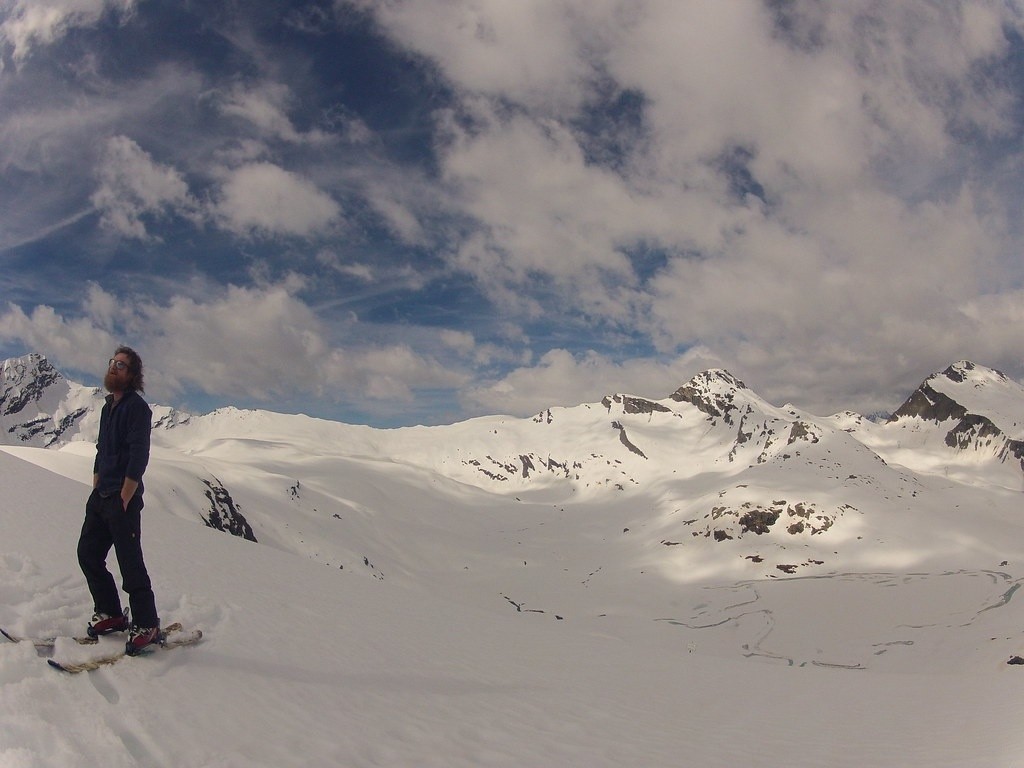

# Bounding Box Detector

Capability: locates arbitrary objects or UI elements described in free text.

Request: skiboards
[0,622,202,674]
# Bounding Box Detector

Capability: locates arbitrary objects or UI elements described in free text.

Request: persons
[77,345,159,649]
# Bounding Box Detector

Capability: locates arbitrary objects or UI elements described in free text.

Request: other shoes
[89,613,160,648]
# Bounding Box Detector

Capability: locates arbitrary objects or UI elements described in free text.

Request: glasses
[109,358,131,370]
